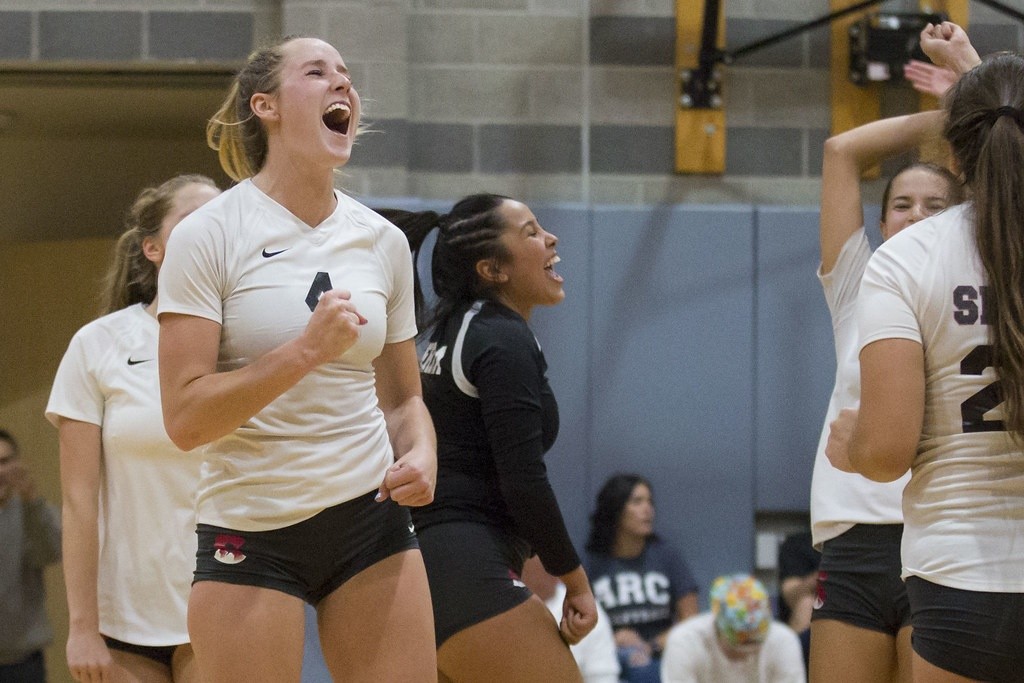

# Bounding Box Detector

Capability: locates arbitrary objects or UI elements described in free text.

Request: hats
[711,573,772,655]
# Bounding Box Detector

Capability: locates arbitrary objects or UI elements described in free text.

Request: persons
[659,571,806,683]
[155,37,440,682]
[518,548,620,683]
[775,528,822,683]
[41,174,220,683]
[824,47,1024,682]
[0,430,62,683]
[582,469,699,683]
[376,187,595,682]
[809,21,981,683]
